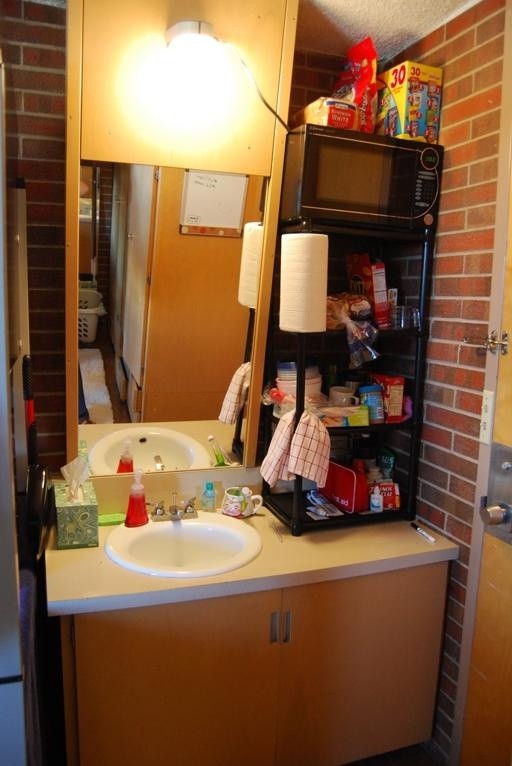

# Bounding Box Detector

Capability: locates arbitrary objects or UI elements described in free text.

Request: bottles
[201,482,217,513]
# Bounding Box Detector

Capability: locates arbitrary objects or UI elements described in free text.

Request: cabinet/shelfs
[267,227,434,541]
[111,164,267,422]
[62,559,448,763]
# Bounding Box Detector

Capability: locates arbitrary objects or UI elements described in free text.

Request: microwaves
[279,123,445,232]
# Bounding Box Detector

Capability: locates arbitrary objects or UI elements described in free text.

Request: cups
[221,485,263,519]
[329,386,360,406]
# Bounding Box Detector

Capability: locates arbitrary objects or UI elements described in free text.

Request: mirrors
[81,161,279,474]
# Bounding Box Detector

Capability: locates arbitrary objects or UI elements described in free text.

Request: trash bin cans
[78,272,103,343]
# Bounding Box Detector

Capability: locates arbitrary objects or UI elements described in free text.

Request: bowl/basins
[274,361,323,399]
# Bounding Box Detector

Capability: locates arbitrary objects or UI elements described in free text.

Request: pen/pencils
[410,520,436,543]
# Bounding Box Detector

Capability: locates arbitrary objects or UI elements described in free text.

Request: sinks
[106,509,264,578]
[90,426,211,475]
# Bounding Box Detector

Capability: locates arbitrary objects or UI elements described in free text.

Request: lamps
[125,20,259,158]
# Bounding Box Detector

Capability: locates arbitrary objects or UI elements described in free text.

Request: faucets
[154,455,165,470]
[170,505,185,521]
